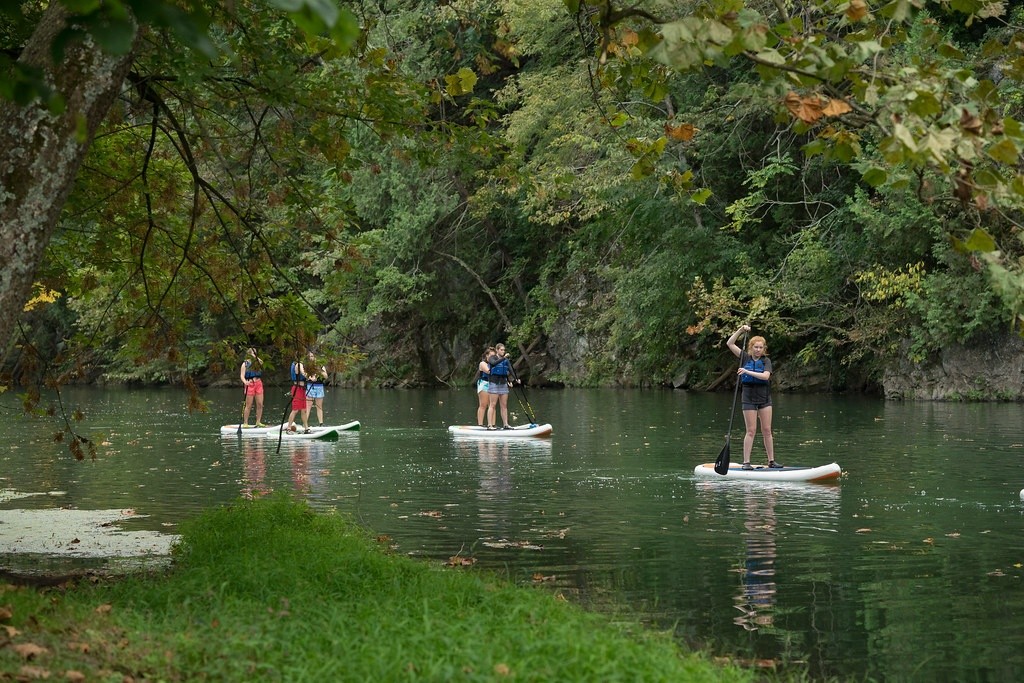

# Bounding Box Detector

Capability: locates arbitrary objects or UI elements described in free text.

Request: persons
[725,325,784,470]
[285,350,329,437]
[239,347,266,430]
[476,343,521,431]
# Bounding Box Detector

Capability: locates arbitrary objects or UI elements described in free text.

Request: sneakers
[768,460,783,468]
[742,463,753,470]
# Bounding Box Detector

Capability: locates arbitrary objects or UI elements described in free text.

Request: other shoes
[244,424,249,428]
[286,429,295,435]
[480,424,484,427]
[319,423,326,426]
[256,422,265,427]
[493,426,501,430]
[304,429,313,434]
[503,425,514,429]
[487,425,497,430]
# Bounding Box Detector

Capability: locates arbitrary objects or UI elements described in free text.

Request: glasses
[499,348,506,351]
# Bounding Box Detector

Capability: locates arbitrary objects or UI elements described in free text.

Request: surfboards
[447,423,555,438]
[694,462,842,482]
[220,420,361,434]
[266,430,339,438]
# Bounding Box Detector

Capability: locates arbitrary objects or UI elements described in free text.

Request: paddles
[506,358,537,426]
[714,330,749,476]
